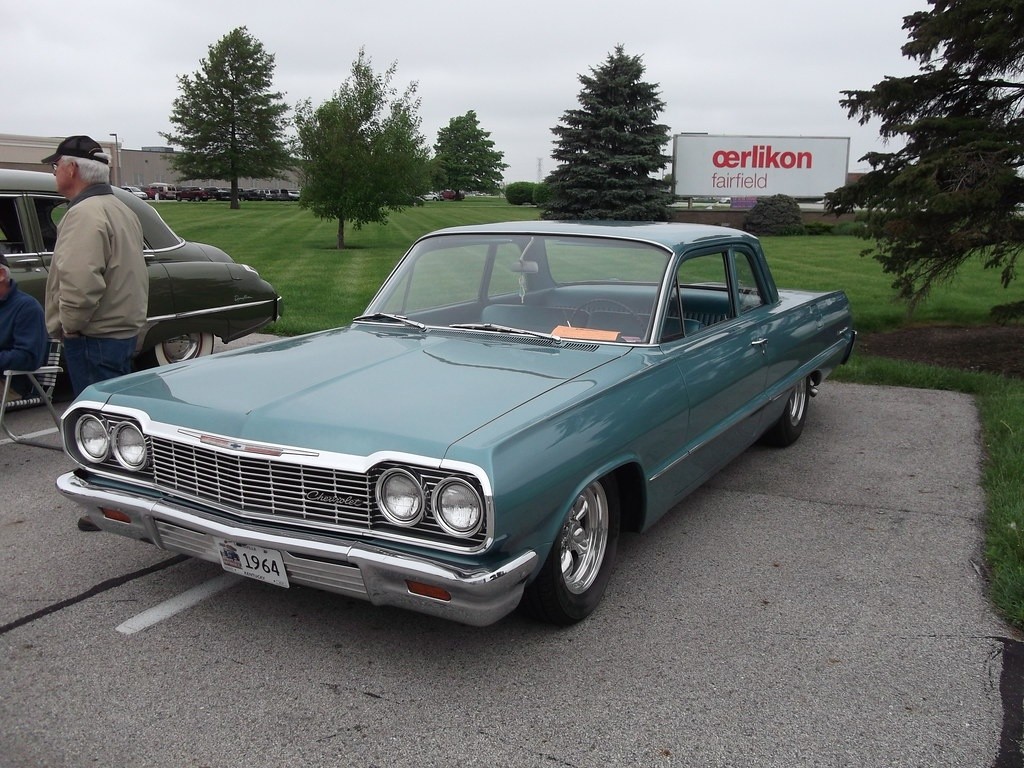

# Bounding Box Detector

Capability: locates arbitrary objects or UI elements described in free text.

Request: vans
[148,182,177,199]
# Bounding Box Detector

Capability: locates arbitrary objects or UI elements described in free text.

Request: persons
[0,253,49,403]
[41,135,149,532]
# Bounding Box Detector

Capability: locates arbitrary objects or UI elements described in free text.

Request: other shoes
[77,515,101,531]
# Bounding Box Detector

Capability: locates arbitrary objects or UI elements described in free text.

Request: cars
[422,191,445,202]
[0,167,283,368]
[175,188,215,202]
[136,185,158,199]
[56,219,860,630]
[719,198,731,203]
[176,185,300,201]
[121,185,148,199]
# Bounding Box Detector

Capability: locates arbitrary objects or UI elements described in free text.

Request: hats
[41,135,109,164]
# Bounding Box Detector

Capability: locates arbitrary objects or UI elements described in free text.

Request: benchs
[667,312,727,326]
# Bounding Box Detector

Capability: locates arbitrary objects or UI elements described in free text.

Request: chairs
[481,304,590,334]
[0,339,65,451]
[587,312,703,338]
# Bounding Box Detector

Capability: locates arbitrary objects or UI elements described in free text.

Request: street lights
[109,133,121,188]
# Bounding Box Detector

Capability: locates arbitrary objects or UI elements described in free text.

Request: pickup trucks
[441,191,465,201]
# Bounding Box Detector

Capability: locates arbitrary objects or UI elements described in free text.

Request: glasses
[51,163,67,171]
